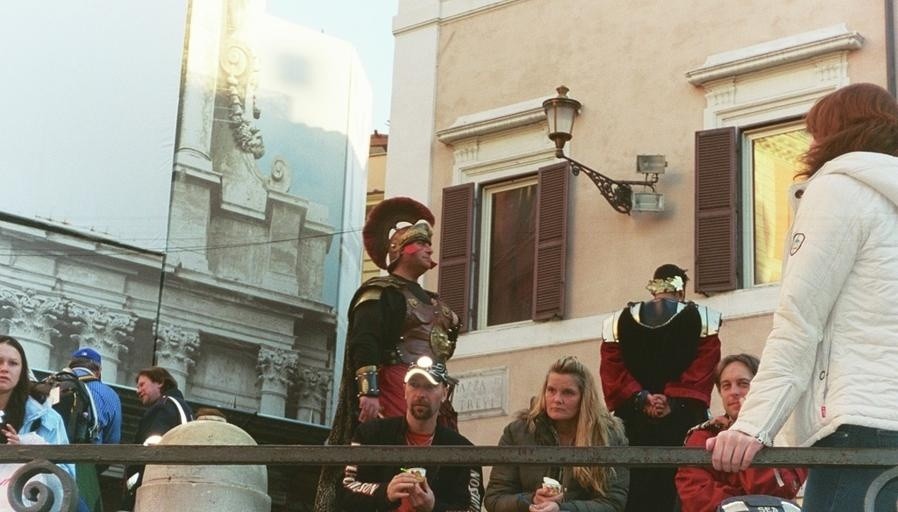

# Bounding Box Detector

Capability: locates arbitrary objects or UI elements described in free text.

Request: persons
[598,263,723,512]
[0,334,93,512]
[334,354,486,512]
[706,82,898,512]
[114,366,194,512]
[40,347,122,512]
[310,195,463,512]
[674,353,807,512]
[481,350,631,512]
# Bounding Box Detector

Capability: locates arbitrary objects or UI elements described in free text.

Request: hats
[404,359,448,387]
[72,348,101,363]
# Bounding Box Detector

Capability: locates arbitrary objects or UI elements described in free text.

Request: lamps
[542,81,667,218]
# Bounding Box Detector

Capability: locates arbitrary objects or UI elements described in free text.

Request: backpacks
[29,368,100,444]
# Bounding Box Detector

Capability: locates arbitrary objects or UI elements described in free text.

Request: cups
[406,467,427,484]
[543,482,561,494]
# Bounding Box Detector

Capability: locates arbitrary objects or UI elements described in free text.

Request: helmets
[363,197,435,273]
